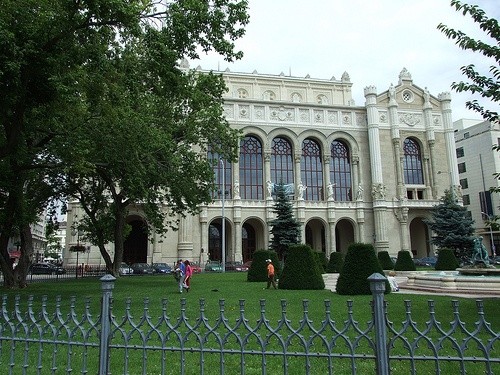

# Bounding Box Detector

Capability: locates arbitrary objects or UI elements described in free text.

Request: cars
[32,262,66,275]
[414,256,500,267]
[120,261,250,276]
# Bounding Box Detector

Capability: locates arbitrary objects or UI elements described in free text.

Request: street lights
[71,230,84,277]
[219,156,225,272]
[481,211,496,257]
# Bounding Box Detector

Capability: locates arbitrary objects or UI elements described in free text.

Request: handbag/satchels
[269,274,274,279]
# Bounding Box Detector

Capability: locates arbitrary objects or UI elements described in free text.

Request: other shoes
[265,288,269,290]
[274,288,277,290]
[177,292,182,294]
[186,287,190,292]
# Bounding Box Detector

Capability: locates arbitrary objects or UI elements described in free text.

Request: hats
[389,271,396,276]
[265,259,272,263]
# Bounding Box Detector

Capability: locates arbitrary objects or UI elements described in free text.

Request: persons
[357,183,363,199]
[51,260,64,266]
[297,181,307,198]
[326,182,335,199]
[266,180,273,197]
[371,183,387,199]
[457,184,464,200]
[403,183,406,197]
[265,259,277,290]
[473,236,484,261]
[234,180,240,198]
[174,259,192,294]
